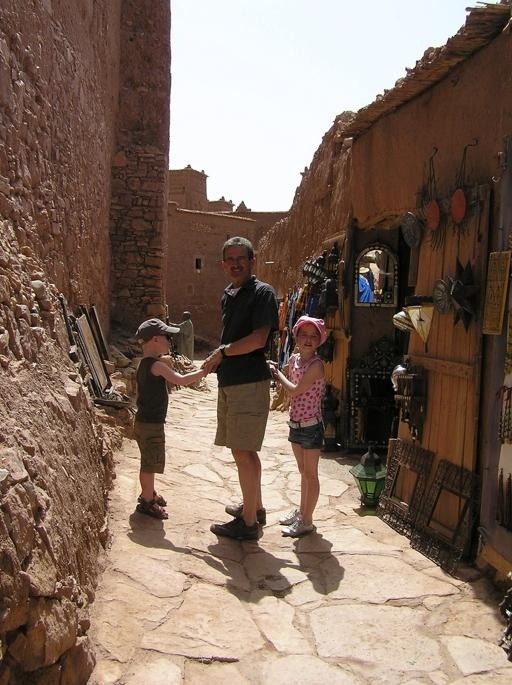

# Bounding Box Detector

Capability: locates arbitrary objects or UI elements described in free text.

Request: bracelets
[219,343,227,358]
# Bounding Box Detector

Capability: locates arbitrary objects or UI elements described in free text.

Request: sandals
[136,497,168,519]
[138,490,167,506]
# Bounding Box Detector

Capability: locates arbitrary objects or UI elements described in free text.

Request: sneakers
[279,509,302,525]
[226,503,267,525]
[210,515,263,539]
[281,519,314,536]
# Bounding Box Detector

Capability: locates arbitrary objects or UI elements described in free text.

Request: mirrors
[353,243,398,308]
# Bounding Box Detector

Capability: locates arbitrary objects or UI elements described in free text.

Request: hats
[359,267,369,274]
[292,316,328,346]
[135,318,181,346]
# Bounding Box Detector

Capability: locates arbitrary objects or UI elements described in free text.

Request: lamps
[349,444,390,511]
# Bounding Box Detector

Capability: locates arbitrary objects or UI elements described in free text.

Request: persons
[264,315,328,536]
[200,235,280,541]
[133,318,208,521]
[168,310,196,364]
[358,265,375,302]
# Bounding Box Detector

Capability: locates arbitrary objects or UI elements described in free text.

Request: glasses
[158,333,172,340]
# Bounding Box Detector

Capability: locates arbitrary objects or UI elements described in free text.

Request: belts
[286,418,322,429]
